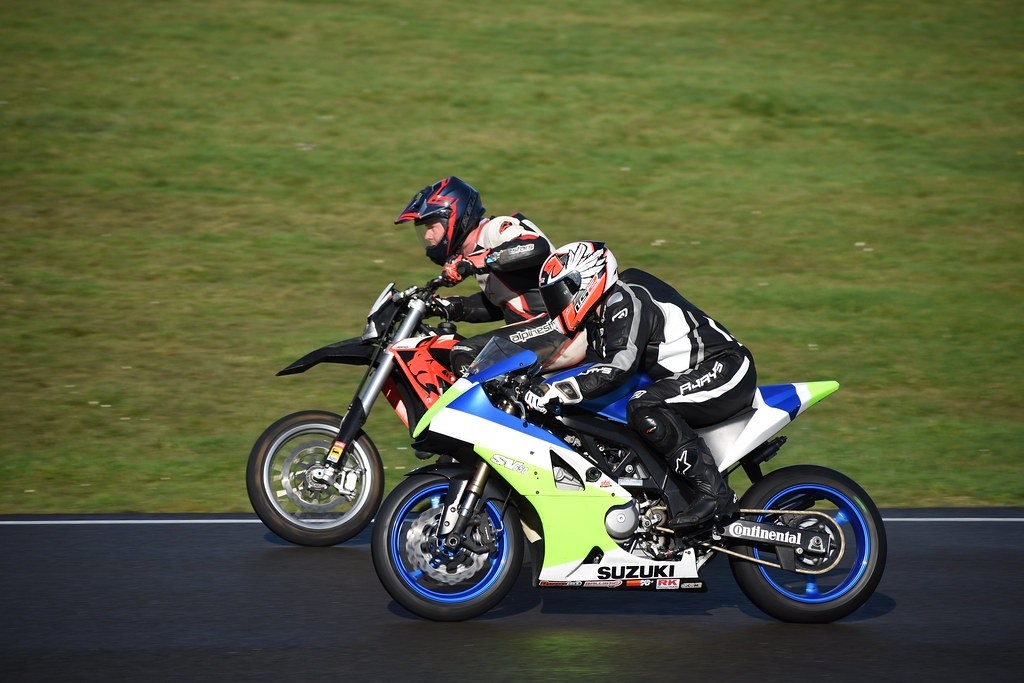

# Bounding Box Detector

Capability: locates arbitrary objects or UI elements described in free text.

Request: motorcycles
[369,325,890,623]
[244,260,580,548]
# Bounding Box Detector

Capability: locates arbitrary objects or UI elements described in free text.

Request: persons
[523,240,757,527]
[395,178,559,378]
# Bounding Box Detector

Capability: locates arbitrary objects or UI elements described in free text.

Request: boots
[666,437,740,528]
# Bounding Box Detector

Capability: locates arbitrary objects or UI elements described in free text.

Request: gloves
[440,248,490,287]
[524,375,583,414]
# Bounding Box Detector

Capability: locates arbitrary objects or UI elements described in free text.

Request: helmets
[539,240,619,338]
[394,177,486,267]
